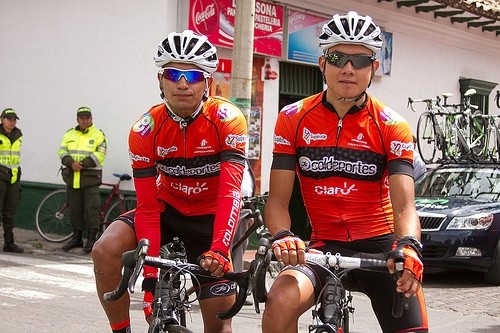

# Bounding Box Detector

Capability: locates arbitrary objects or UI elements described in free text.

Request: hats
[77,107,91,117]
[1,109,19,120]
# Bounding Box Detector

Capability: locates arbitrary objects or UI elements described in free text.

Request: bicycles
[103,192,409,333]
[35,172,138,243]
[406,89,500,164]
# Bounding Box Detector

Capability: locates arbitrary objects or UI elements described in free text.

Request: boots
[3,232,23,253]
[62,230,82,249]
[82,229,96,251]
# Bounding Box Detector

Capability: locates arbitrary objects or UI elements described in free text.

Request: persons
[264,11,429,333]
[57,106,107,253]
[0,108,25,254]
[89,30,262,333]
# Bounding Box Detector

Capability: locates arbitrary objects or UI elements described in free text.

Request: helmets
[154,29,220,73]
[318,10,383,54]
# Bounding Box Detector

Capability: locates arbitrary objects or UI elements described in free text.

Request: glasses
[159,67,209,83]
[323,51,374,68]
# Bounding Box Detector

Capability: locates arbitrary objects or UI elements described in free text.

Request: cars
[414,161,500,285]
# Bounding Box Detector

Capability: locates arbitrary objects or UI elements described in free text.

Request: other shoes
[243,301,252,305]
